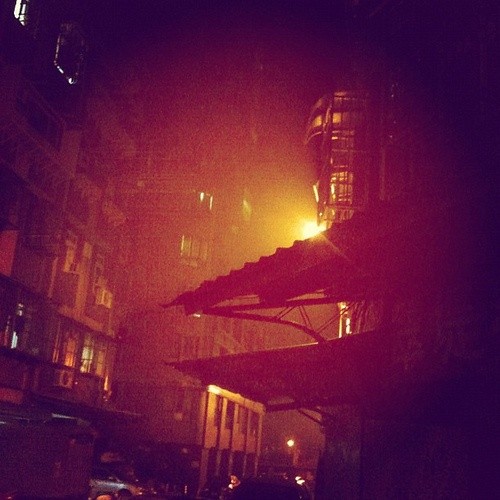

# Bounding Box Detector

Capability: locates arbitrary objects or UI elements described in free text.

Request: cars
[89,463,143,500]
[224,471,315,500]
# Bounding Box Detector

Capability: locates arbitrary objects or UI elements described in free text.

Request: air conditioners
[52,369,73,388]
[95,287,113,308]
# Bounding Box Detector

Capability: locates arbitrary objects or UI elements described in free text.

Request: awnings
[162,209,415,412]
